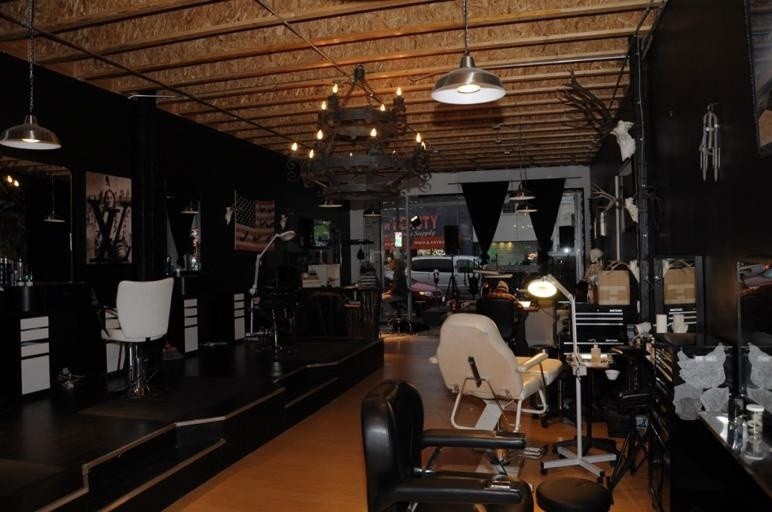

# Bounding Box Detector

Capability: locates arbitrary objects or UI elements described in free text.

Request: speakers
[444,225,460,255]
[560,227,574,248]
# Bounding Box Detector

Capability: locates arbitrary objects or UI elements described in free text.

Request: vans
[409,253,489,299]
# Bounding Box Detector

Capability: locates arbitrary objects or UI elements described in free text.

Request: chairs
[436,296,636,457]
[89,275,178,402]
[361,378,533,512]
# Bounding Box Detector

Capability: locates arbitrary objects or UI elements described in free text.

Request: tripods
[609,361,648,488]
[442,254,462,305]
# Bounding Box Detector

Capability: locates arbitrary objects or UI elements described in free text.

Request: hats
[497,280,509,288]
[590,248,603,263]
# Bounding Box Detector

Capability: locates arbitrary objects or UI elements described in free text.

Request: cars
[382,272,442,300]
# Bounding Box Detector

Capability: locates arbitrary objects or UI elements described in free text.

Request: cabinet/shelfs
[225,290,255,347]
[97,304,137,377]
[166,293,200,356]
[14,312,53,404]
[645,325,733,511]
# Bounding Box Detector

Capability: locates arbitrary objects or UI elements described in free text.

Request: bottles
[588,339,602,366]
[1,257,34,288]
[745,404,765,460]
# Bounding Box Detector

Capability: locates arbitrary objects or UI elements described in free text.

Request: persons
[96,189,131,263]
[485,280,523,323]
[381,259,408,324]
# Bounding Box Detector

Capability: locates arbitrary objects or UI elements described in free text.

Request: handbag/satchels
[574,280,590,302]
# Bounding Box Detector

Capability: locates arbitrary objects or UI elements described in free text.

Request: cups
[673,314,684,334]
[656,314,668,334]
[605,370,620,380]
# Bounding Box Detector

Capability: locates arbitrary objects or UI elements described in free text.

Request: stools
[390,300,408,327]
[536,477,614,510]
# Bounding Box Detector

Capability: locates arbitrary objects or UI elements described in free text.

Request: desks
[695,410,772,501]
[292,282,383,349]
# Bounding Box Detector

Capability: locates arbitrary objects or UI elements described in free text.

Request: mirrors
[652,255,706,335]
[160,193,205,276]
[0,155,75,285]
[733,255,772,428]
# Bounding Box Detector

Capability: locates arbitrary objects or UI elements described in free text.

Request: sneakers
[383,308,402,326]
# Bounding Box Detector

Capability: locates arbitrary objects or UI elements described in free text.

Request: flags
[235,192,276,253]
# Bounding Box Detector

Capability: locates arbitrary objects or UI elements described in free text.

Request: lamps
[427,1,508,108]
[244,227,297,344]
[282,62,435,214]
[1,0,62,152]
[514,148,540,214]
[509,120,538,202]
[526,274,621,484]
[390,200,403,250]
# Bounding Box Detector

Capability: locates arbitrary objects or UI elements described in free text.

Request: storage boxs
[661,266,697,306]
